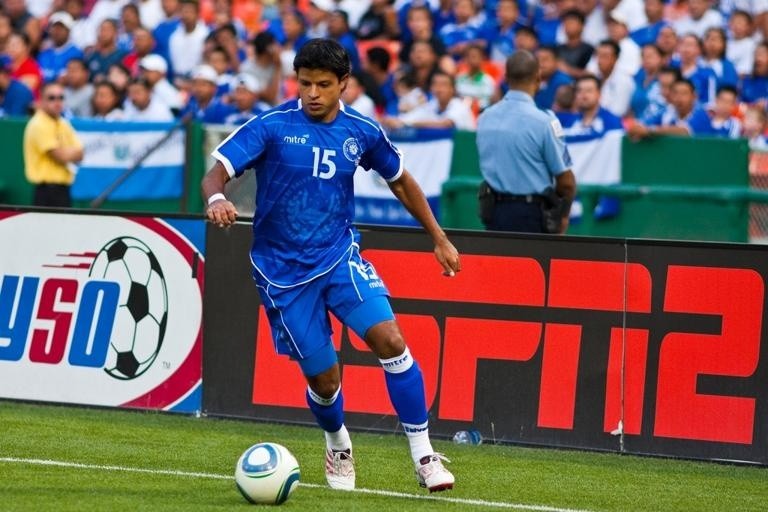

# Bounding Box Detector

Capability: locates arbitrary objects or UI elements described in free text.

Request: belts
[497,194,551,202]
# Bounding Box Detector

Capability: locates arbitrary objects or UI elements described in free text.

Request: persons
[556,74,625,222]
[198,38,463,493]
[0,0,767,153]
[473,48,577,235]
[21,81,86,207]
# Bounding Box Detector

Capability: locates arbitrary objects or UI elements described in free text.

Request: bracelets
[206,192,225,207]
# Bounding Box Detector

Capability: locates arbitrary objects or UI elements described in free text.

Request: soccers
[235,442,300,505]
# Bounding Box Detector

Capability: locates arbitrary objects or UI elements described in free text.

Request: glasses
[47,95,65,101]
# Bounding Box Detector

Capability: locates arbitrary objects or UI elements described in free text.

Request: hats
[49,11,74,31]
[138,53,168,74]
[192,64,217,84]
[229,73,259,96]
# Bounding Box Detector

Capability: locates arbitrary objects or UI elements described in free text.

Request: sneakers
[325,445,355,490]
[415,452,455,492]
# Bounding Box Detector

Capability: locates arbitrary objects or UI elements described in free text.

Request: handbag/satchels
[478,181,496,224]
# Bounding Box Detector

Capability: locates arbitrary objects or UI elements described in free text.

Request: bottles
[453,430,483,447]
